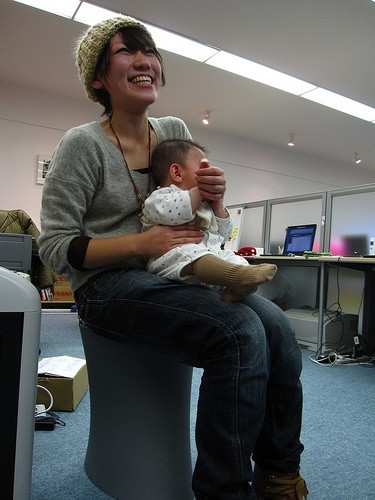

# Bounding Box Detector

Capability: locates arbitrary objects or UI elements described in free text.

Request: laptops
[260,223,317,256]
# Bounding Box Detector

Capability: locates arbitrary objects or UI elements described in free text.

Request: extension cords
[335,354,370,363]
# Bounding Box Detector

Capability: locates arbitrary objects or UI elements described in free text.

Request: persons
[141,139,277,302]
[37,16,309,500]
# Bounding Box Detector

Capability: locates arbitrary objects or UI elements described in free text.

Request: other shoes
[257,473,310,500]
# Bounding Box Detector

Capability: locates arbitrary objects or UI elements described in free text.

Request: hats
[75,18,150,104]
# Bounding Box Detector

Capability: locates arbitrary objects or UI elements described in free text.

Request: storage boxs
[54,281,75,301]
[37,356,89,411]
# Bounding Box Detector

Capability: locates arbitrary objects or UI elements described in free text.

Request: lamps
[288,136,295,147]
[354,152,361,164]
[203,113,209,125]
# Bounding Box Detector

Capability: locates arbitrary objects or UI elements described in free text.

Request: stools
[79,324,194,500]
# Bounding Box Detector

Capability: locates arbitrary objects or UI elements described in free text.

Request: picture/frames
[37,154,52,184]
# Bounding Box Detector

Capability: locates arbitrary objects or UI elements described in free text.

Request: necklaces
[110,119,152,217]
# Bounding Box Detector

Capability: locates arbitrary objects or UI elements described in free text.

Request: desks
[242,255,375,359]
